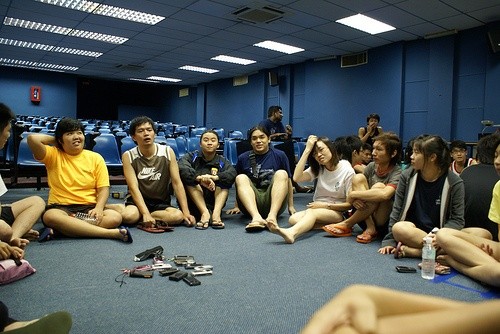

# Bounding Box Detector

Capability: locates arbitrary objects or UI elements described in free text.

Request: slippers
[394,241,409,259]
[356,230,377,243]
[119,226,133,243]
[244,221,267,232]
[211,219,225,229]
[195,220,210,230]
[136,219,175,233]
[322,224,353,237]
[37,227,55,242]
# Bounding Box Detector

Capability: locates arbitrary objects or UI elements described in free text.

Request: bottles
[110,191,127,198]
[421,236,436,280]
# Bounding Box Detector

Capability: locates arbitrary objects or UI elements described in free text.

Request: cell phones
[395,265,418,273]
[158,255,213,286]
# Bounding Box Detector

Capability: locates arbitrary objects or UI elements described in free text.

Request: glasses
[451,148,465,154]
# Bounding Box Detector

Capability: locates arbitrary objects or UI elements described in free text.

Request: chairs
[4,115,312,190]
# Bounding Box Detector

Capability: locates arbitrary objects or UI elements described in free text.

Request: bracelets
[328,202,331,208]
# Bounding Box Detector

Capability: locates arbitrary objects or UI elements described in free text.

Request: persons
[226,126,296,231]
[258,105,312,194]
[267,134,356,243]
[27,117,134,245]
[301,282,500,334]
[178,129,237,230]
[103,116,197,227]
[358,113,385,161]
[324,125,500,299]
[0,101,45,260]
[0,301,73,334]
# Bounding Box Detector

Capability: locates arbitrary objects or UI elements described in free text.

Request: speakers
[269,70,278,87]
